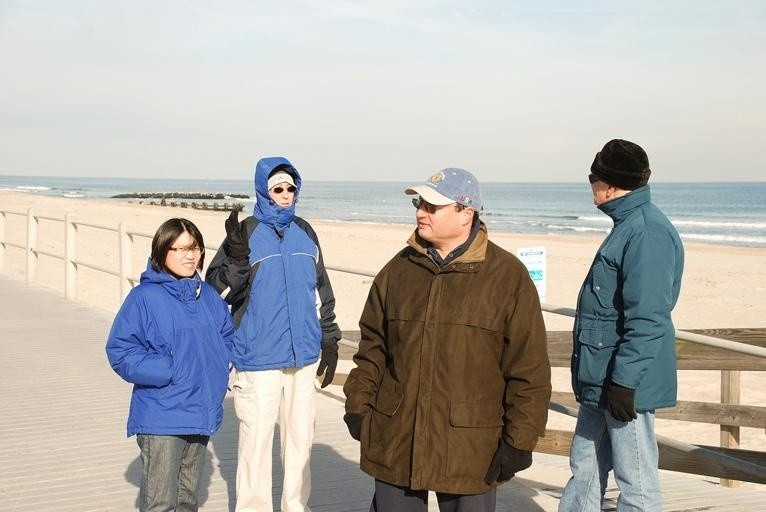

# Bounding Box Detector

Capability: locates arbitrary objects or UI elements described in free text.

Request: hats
[404,168,481,212]
[268,171,297,192]
[591,139,651,190]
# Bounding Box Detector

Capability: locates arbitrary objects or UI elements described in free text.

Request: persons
[559,141,684,511]
[204,158,342,512]
[104,215,237,512]
[343,168,553,512]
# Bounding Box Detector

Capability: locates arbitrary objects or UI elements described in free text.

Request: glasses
[274,186,296,193]
[412,198,452,214]
[170,247,205,255]
[589,175,599,184]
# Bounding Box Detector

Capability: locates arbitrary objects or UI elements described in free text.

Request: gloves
[343,413,363,442]
[607,382,637,422]
[225,211,250,260]
[485,439,532,486]
[317,339,338,388]
